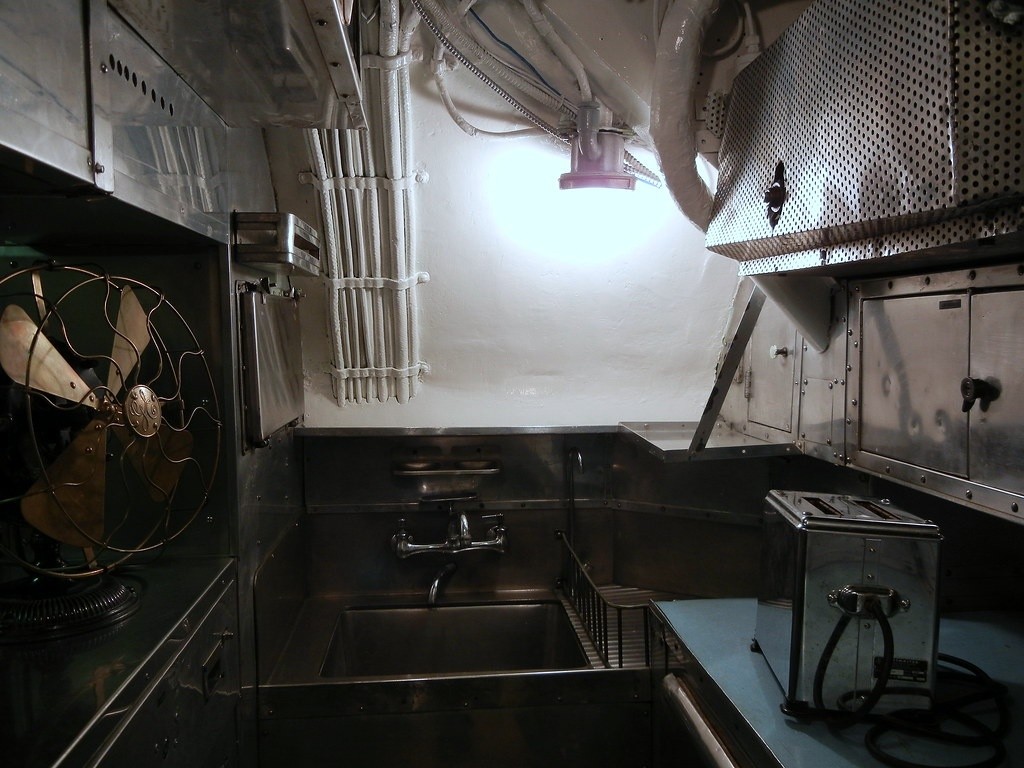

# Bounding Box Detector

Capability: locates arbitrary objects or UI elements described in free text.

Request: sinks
[312,588,588,685]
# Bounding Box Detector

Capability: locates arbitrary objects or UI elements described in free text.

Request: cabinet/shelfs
[740,283,803,442]
[846,263,1024,525]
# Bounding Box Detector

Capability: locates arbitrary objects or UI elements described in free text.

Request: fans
[0,264,222,645]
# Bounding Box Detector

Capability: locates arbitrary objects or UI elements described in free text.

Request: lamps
[555,130,637,191]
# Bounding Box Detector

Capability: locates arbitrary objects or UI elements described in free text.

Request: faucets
[424,512,474,610]
